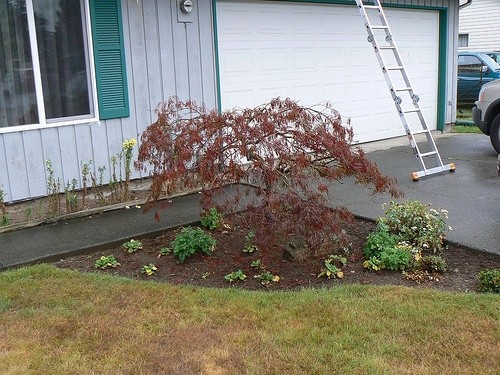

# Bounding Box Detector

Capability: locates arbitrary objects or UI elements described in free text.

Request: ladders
[355,0,455,181]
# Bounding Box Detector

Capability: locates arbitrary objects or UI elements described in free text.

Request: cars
[457,52,500,103]
[471,79,500,155]
